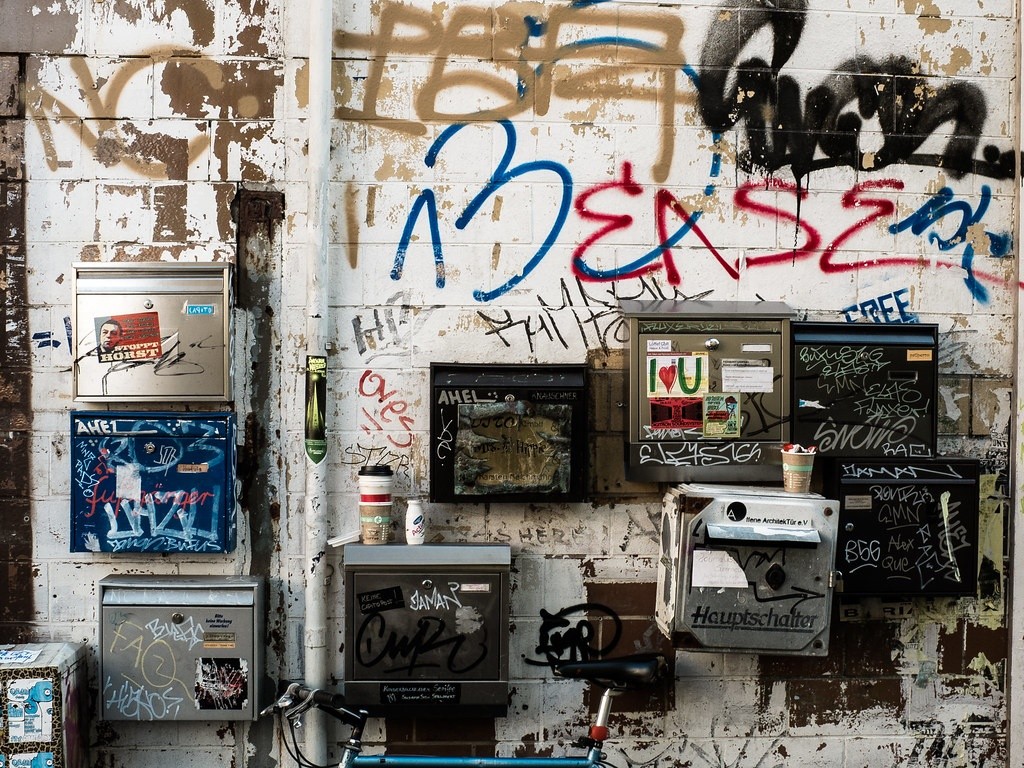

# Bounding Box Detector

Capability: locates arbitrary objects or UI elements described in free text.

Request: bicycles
[260,649,675,768]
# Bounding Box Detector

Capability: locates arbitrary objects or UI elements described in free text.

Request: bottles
[405,498,426,545]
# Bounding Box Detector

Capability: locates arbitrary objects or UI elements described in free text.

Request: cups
[781,448,816,493]
[357,464,394,504]
[357,501,395,544]
